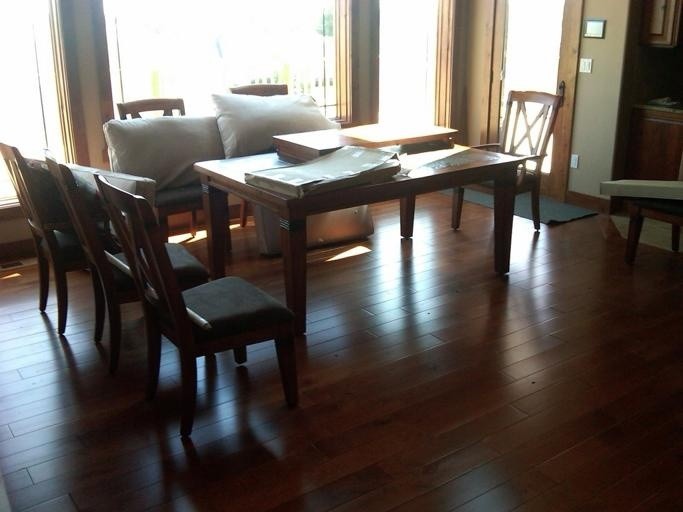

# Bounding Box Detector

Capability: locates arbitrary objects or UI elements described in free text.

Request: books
[244,146,402,201]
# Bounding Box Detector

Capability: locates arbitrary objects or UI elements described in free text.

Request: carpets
[442,184,600,225]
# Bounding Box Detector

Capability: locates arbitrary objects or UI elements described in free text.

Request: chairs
[450,89,562,241]
[114,99,234,254]
[94,170,299,438]
[2,144,124,336]
[44,156,213,372]
[230,83,290,227]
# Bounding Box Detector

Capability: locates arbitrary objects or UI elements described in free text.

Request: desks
[193,133,522,341]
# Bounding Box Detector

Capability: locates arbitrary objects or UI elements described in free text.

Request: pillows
[103,113,224,174]
[43,147,152,242]
[210,91,341,160]
[250,201,375,260]
